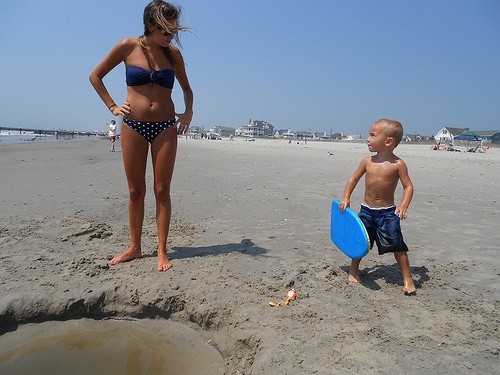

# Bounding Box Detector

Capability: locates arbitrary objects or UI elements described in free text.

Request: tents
[452,131,482,153]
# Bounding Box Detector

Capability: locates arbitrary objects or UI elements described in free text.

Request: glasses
[158,25,179,36]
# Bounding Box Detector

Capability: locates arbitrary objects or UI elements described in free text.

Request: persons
[107,120,117,152]
[433,142,441,150]
[88,0,193,271]
[480,146,489,153]
[443,143,454,151]
[338,118,416,294]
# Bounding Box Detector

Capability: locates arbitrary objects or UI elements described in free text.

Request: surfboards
[330,201,372,260]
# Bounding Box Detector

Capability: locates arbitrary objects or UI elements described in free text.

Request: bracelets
[108,103,116,110]
